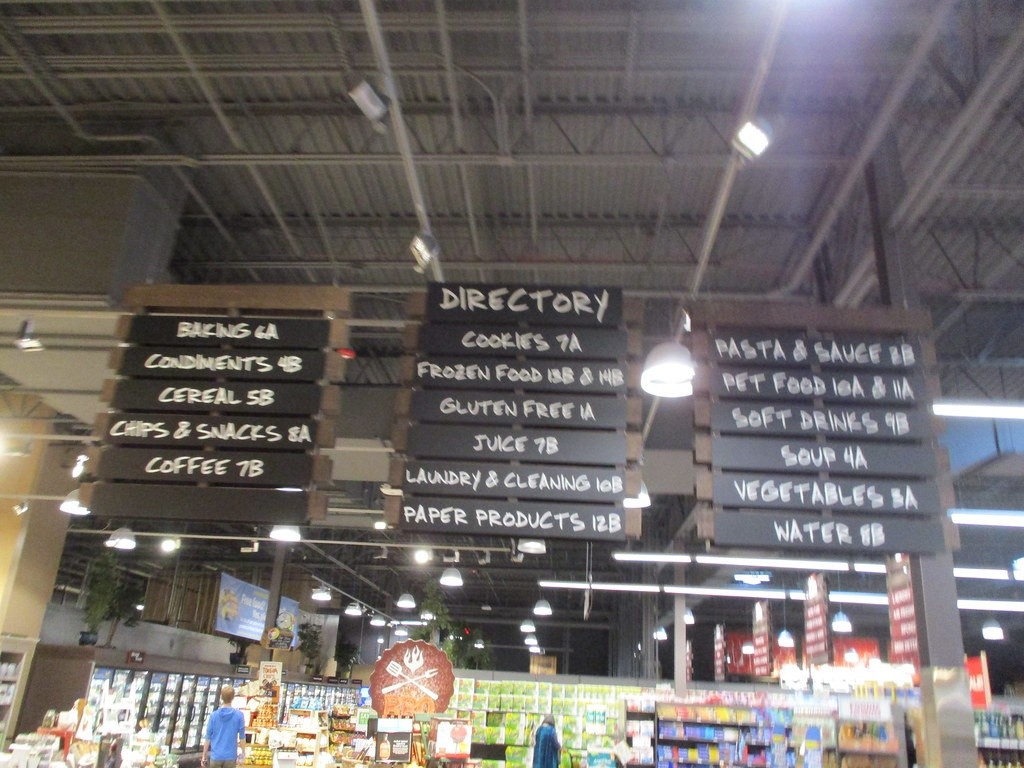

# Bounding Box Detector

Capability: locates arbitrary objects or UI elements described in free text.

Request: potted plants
[228,634,253,665]
[80,551,122,646]
[334,634,358,678]
[296,623,327,675]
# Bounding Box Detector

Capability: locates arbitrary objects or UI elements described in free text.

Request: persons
[201,687,245,768]
[533,713,562,768]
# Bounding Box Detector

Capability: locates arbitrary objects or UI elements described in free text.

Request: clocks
[276,611,294,630]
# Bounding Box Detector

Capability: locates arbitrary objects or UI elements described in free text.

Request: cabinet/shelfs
[443,667,906,768]
[0,633,38,752]
[75,659,363,768]
[910,705,1024,768]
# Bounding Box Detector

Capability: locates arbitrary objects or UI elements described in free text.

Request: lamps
[732,111,790,161]
[508,457,1024,654]
[270,523,301,543]
[411,226,440,269]
[13,317,43,354]
[348,71,392,120]
[13,500,31,516]
[312,517,491,663]
[59,488,92,515]
[105,519,137,548]
[640,308,698,398]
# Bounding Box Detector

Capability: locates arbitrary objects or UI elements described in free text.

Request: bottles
[973,711,1024,740]
[380,734,390,760]
[252,704,278,727]
[981,750,1023,768]
[245,747,272,766]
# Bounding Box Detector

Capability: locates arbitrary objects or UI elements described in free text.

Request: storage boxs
[434,675,794,768]
[368,719,412,763]
